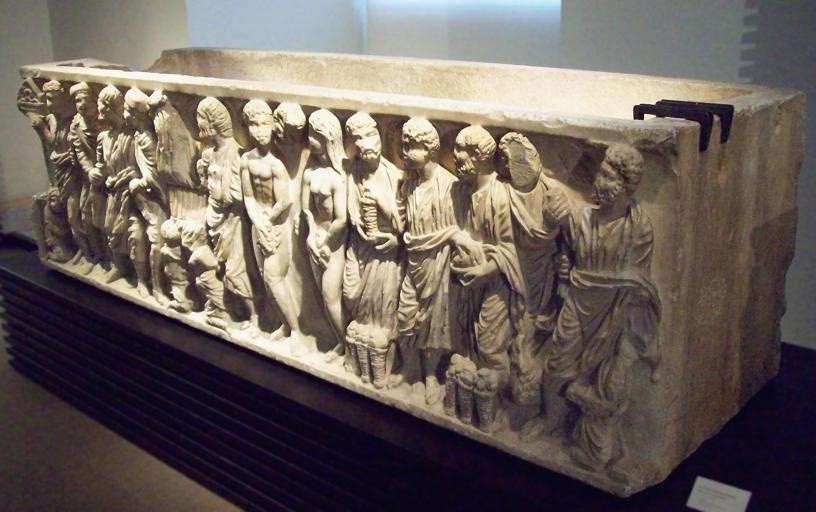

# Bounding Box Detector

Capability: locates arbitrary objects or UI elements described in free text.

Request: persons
[23,80,663,469]
